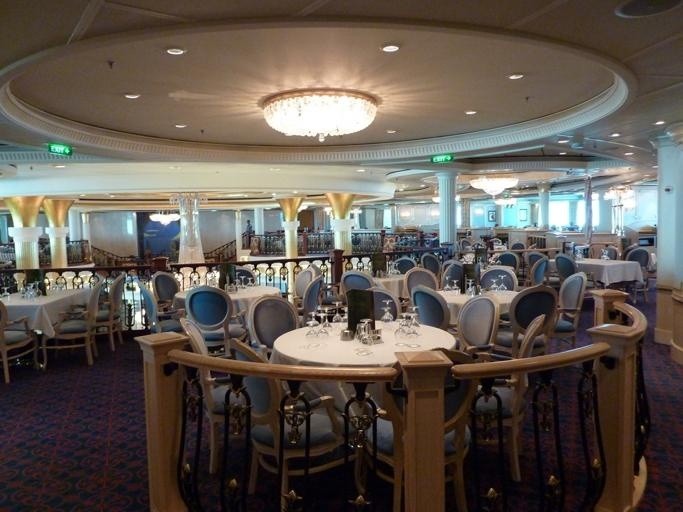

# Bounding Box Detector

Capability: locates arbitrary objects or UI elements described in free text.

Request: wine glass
[0,280,59,304]
[189,274,255,294]
[304,296,423,358]
[442,275,507,300]
[573,248,609,263]
[462,254,502,270]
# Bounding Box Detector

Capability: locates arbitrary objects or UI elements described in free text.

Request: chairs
[43,278,107,363]
[136,225,662,512]
[76,274,128,350]
[0,301,42,384]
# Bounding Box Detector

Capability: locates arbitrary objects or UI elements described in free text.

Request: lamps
[469,177,519,198]
[148,208,180,228]
[257,87,380,145]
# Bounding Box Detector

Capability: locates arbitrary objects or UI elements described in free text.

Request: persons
[246,219,252,232]
